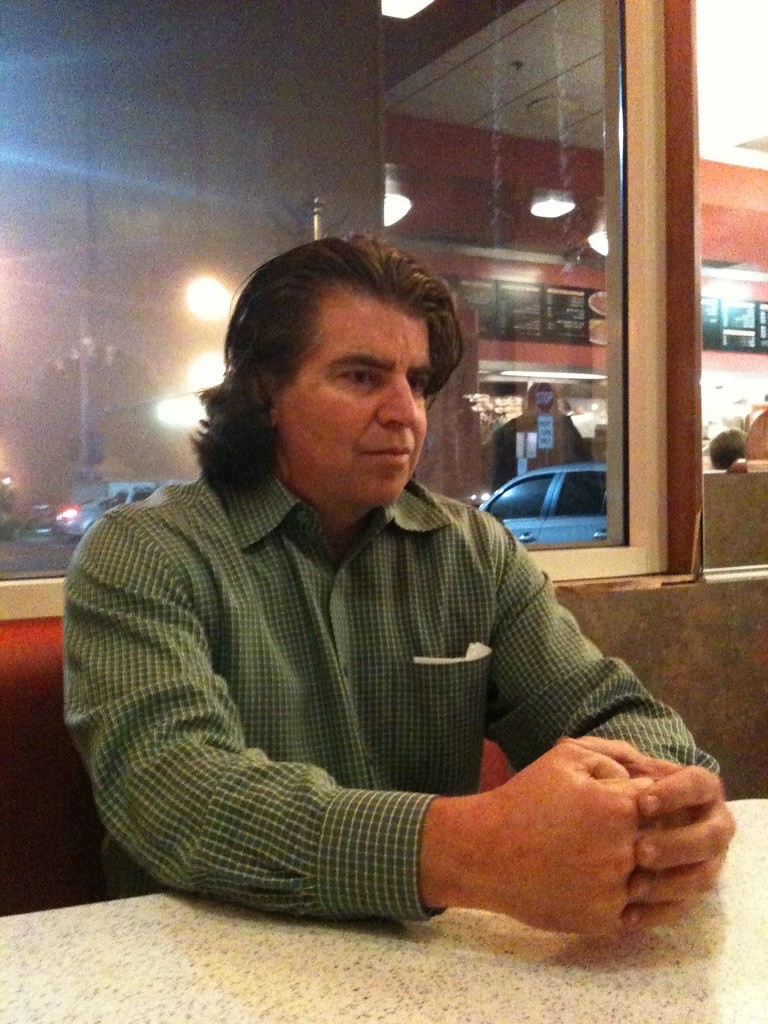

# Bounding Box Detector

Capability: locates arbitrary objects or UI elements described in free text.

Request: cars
[470,460,610,548]
[50,487,155,541]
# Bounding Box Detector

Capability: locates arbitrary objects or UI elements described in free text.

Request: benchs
[0,616,510,913]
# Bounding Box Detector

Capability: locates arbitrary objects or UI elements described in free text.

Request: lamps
[531,183,576,219]
[588,202,608,254]
[384,161,413,227]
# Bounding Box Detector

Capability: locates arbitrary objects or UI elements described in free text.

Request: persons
[62,235,737,932]
[491,386,596,495]
[709,429,749,470]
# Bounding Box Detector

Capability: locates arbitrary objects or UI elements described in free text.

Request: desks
[0,799,768,1024]
